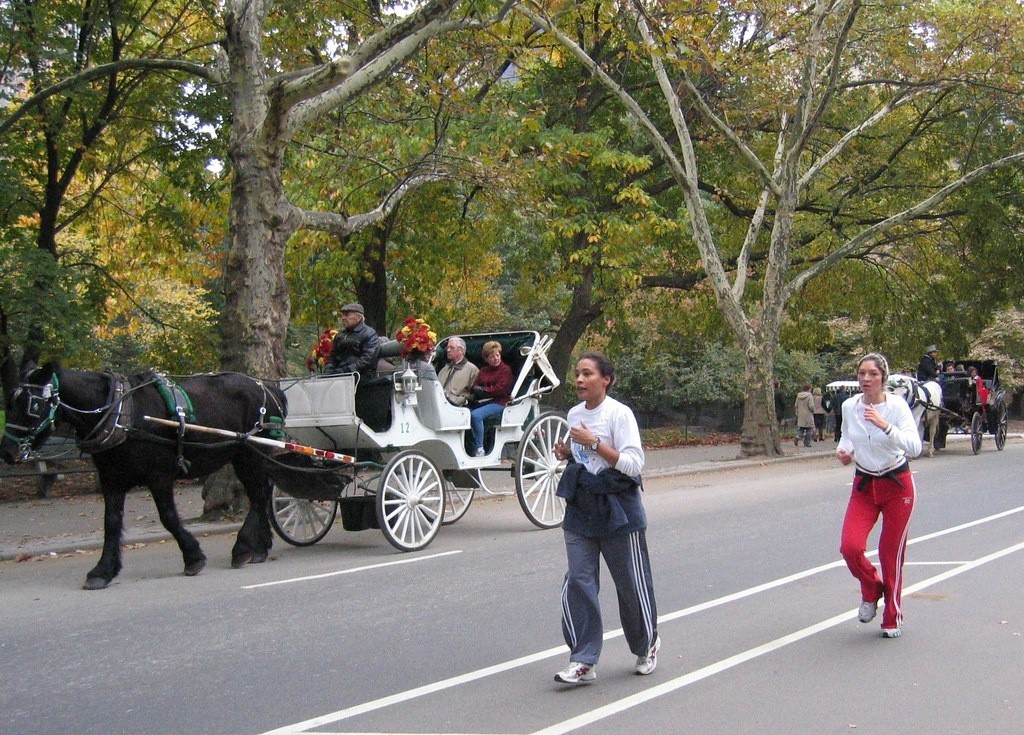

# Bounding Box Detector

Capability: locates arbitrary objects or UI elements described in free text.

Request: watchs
[590,436,601,451]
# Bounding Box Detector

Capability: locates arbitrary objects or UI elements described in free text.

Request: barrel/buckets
[338,477,403,532]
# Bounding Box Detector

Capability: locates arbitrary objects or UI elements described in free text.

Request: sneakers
[858,600,878,623]
[634,636,660,675]
[879,626,903,637]
[554,661,596,684]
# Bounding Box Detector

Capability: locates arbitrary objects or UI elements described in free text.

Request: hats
[340,304,365,314]
[925,345,937,355]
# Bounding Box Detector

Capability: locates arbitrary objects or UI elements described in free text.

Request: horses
[886,380,942,458]
[0,346,287,591]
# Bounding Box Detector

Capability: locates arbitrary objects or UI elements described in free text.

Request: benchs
[484,377,539,428]
[415,379,471,432]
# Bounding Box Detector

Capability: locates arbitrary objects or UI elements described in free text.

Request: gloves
[325,363,337,374]
[471,385,483,394]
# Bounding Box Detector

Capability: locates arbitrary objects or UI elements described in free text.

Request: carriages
[0,330,569,591]
[887,359,1008,455]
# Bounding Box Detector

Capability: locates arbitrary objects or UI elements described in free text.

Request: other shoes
[793,437,799,446]
[472,448,486,457]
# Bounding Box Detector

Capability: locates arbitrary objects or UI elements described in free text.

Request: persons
[918,344,983,403]
[321,303,380,389]
[466,341,514,457]
[794,385,851,447]
[835,352,922,637]
[438,337,479,407]
[554,351,661,683]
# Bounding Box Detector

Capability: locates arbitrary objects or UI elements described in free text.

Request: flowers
[306,328,339,374]
[396,316,437,360]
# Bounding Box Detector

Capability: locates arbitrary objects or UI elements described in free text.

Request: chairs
[357,338,403,392]
